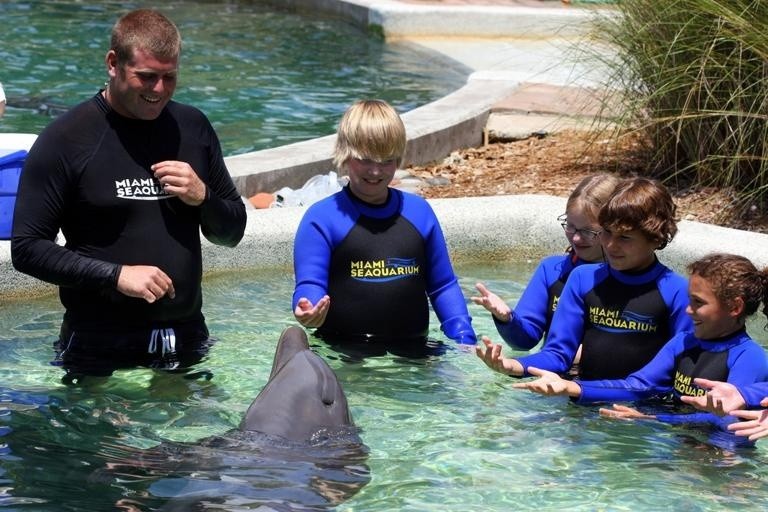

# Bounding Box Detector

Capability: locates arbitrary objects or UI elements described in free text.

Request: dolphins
[145,324,372,512]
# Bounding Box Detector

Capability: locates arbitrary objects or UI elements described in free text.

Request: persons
[678,375,768,446]
[289,97,480,372]
[474,176,699,418]
[508,250,768,466]
[7,8,249,399]
[468,170,618,354]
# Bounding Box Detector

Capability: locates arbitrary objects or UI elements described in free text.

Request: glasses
[561,218,603,240]
